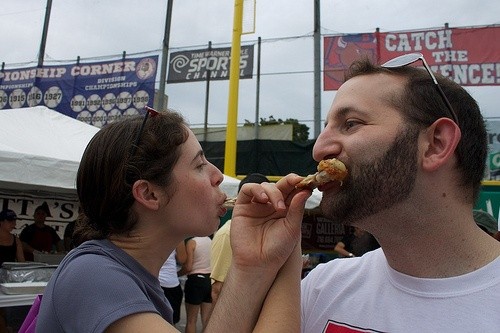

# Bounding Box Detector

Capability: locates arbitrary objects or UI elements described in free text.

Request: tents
[0,102,321,211]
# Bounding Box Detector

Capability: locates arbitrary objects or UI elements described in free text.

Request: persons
[247,57,500,333]
[207,172,279,315]
[183,233,219,333]
[334,224,373,257]
[20,205,63,261]
[154,239,186,326]
[0,208,24,263]
[63,206,91,254]
[469,208,500,242]
[37,112,302,332]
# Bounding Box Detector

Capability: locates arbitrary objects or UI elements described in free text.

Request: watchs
[348,253,354,257]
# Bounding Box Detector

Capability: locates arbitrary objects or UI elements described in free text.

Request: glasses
[123,106,162,189]
[380,52,460,129]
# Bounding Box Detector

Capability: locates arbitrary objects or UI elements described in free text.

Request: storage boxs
[1,282,49,295]
[33,251,66,264]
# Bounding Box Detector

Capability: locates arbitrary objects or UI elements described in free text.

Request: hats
[473,209,498,234]
[0,209,21,220]
